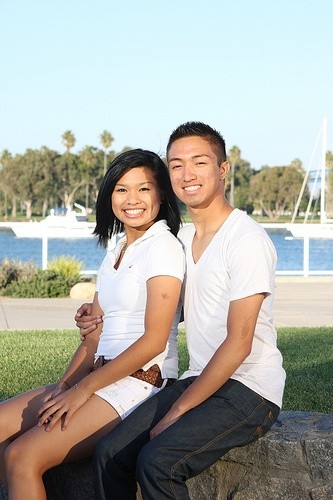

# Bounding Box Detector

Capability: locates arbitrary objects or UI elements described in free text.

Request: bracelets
[75,384,89,401]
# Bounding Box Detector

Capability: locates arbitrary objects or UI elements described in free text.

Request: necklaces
[120,245,126,254]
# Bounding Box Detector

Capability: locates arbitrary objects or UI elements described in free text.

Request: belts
[92,355,165,389]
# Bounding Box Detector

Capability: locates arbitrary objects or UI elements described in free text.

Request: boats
[8,203,100,239]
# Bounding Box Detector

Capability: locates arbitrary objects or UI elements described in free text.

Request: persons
[0,148,187,500]
[74,121,287,500]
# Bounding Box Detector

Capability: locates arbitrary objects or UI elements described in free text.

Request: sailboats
[285,115,333,238]
